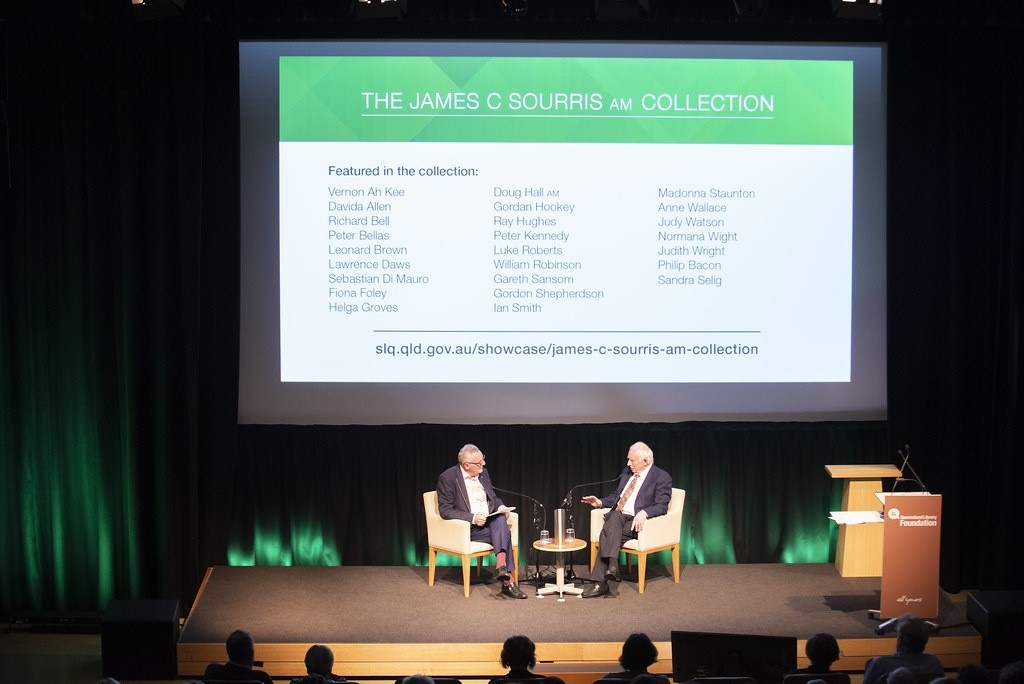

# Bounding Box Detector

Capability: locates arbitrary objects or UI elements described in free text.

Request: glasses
[463,455,486,466]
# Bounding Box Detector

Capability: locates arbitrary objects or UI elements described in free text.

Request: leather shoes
[501,582,527,599]
[605,565,621,583]
[493,565,510,581]
[581,583,610,598]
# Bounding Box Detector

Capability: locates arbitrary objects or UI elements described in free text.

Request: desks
[533,538,588,601]
[824,463,903,577]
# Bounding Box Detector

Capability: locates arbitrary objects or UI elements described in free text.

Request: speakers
[671,631,797,684]
[101,598,179,681]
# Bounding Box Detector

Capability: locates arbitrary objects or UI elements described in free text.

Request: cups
[540,530,550,545]
[565,528,575,543]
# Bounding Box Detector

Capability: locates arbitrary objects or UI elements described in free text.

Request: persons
[580,442,673,598]
[204,628,671,684]
[789,617,1024,684]
[437,444,528,599]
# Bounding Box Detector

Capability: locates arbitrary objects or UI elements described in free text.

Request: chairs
[422,489,519,598]
[591,487,686,593]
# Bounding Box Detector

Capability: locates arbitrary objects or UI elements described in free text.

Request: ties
[615,475,639,511]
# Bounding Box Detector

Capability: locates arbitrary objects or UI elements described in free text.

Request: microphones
[891,444,927,492]
[487,481,544,507]
[563,474,623,503]
